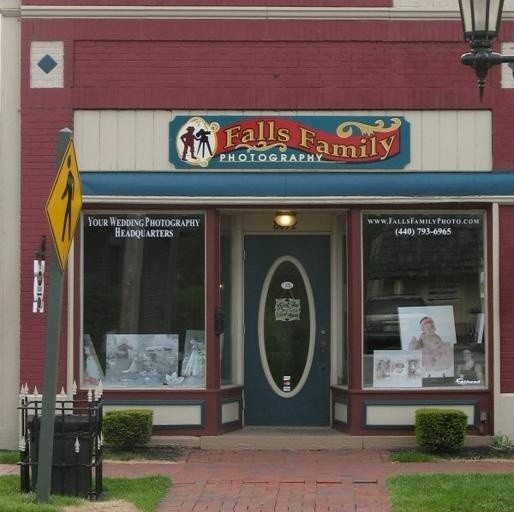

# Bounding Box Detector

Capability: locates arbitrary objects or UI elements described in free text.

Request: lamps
[273,211,297,227]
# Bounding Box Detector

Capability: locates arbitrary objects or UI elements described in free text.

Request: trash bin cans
[26,415,96,496]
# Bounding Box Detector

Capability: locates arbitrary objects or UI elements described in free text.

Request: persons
[411,315,450,372]
[455,347,484,384]
